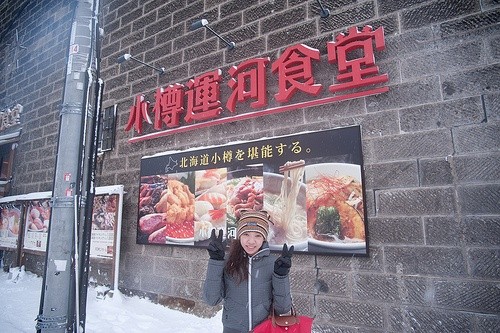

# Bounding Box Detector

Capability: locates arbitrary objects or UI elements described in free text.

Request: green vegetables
[314,205,340,239]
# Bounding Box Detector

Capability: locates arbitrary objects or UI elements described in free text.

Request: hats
[236,211,270,241]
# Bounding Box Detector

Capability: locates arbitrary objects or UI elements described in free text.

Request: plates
[0,197,116,233]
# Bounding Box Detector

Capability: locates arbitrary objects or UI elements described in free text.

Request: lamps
[115,52,165,76]
[190,19,236,50]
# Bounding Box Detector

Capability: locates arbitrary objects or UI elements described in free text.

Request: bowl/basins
[142,160,366,254]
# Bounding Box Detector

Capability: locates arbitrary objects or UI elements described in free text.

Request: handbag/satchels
[253,299,313,333]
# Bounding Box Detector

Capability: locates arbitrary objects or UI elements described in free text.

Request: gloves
[274,244,294,276]
[207,229,226,261]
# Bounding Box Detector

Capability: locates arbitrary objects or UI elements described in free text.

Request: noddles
[263,160,307,245]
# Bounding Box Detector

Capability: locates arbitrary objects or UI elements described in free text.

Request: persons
[202,209,294,333]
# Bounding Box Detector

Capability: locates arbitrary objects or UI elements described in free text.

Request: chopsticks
[279,160,307,173]
